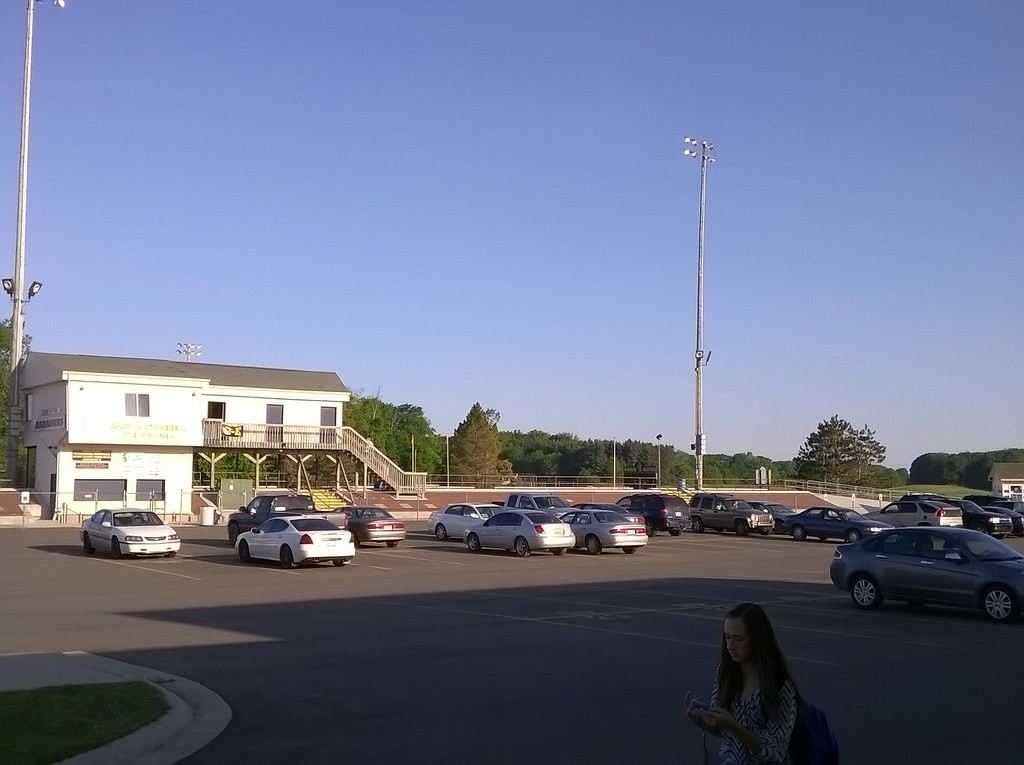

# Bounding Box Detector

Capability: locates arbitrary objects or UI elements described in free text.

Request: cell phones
[691,700,708,711]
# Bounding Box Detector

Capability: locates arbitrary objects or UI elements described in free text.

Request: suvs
[687,492,775,537]
[897,491,1024,539]
[612,492,693,539]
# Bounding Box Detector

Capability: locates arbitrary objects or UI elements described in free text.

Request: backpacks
[717,665,840,765]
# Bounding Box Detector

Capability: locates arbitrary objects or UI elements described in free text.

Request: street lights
[656,433,663,487]
[176,342,204,363]
[683,137,717,494]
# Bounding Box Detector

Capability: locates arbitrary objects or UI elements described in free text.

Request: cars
[330,503,407,549]
[565,503,646,526]
[551,508,649,556]
[830,526,1024,623]
[863,500,964,528]
[427,502,506,541]
[462,509,576,558]
[782,506,897,544]
[235,515,356,570]
[80,507,181,560]
[747,500,794,536]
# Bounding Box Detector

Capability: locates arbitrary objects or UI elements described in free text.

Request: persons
[684,602,804,765]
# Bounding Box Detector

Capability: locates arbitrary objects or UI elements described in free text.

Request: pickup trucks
[481,493,572,521]
[226,493,349,548]
